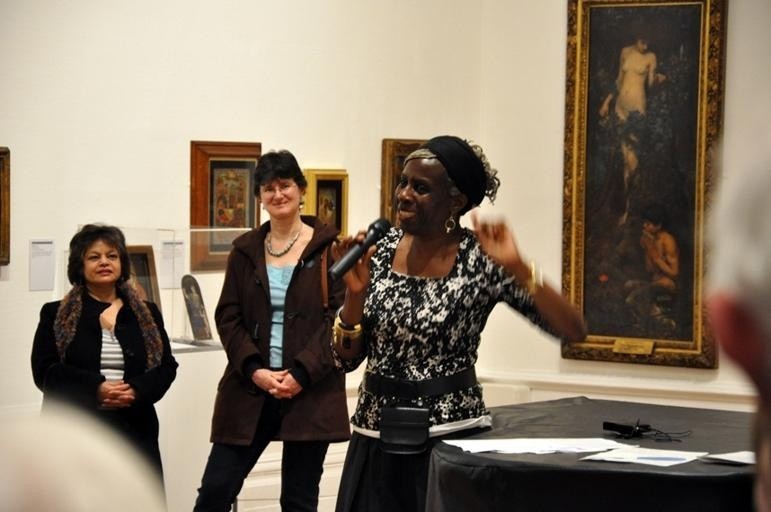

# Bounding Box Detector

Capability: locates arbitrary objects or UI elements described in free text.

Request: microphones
[328,218,391,283]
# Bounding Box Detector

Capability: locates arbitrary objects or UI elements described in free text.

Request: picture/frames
[190,141,261,271]
[125,246,161,314]
[562,1,728,369]
[381,139,429,227]
[301,170,349,241]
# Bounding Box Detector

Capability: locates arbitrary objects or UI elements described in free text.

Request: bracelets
[513,260,541,297]
[330,309,362,346]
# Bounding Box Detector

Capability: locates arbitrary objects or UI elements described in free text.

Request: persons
[598,30,667,182]
[329,133,585,510]
[31,222,179,483]
[700,139,770,512]
[193,149,350,512]
[621,208,680,331]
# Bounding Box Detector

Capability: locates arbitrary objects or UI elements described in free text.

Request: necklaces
[265,225,301,257]
